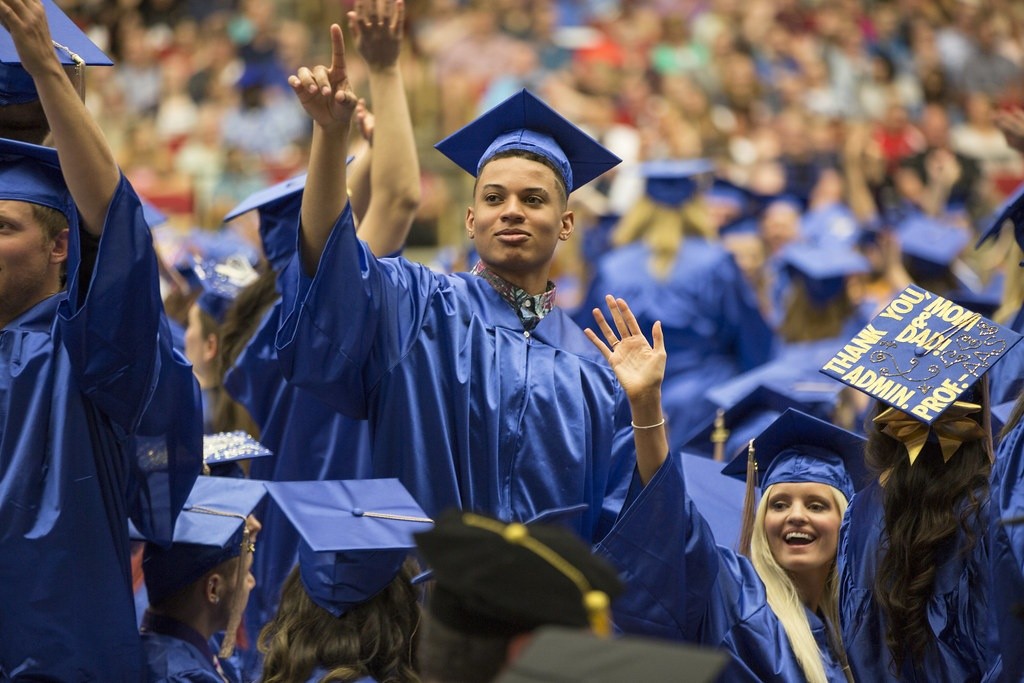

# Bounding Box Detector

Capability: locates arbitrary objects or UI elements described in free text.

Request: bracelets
[631,419,665,428]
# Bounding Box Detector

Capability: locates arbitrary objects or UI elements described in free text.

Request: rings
[611,341,620,348]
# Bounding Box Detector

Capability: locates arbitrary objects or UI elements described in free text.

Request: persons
[0,0,204,683]
[0,0,1024,683]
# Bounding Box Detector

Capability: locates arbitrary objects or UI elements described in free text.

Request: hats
[127,475,268,659]
[0,0,114,106]
[432,90,626,204]
[585,161,1024,499]
[414,509,626,640]
[0,138,74,228]
[263,477,435,619]
[129,59,355,463]
